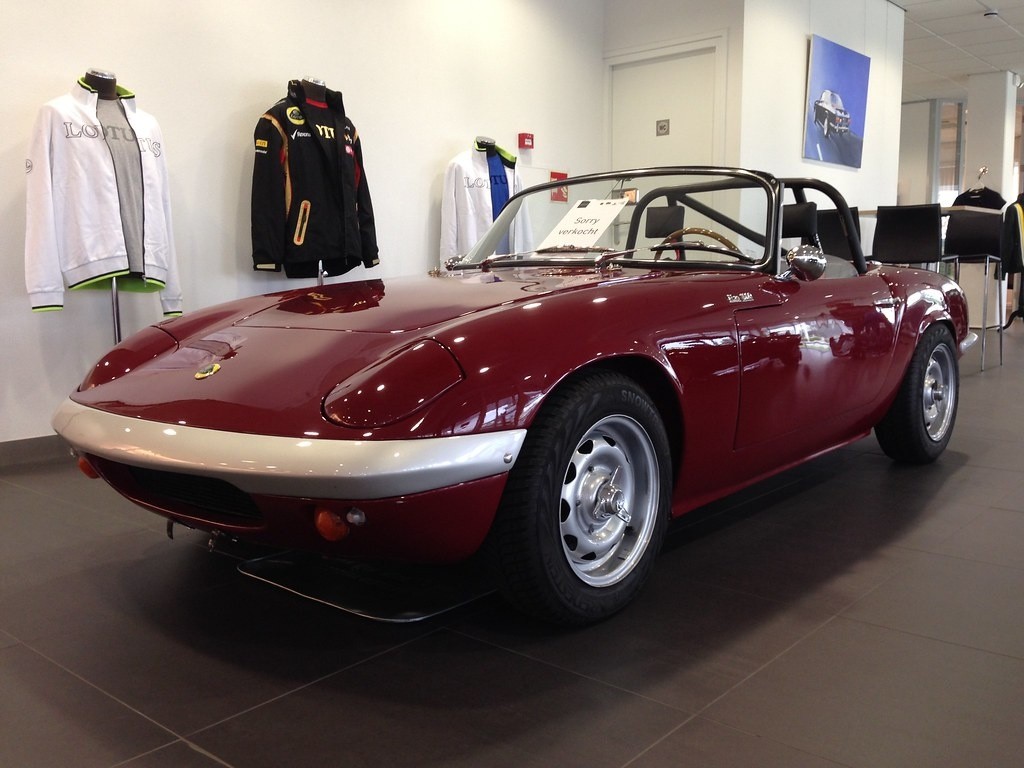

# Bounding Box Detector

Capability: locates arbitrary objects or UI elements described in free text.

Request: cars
[49,166,979,634]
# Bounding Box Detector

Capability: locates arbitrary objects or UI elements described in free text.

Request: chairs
[771,204,1003,373]
